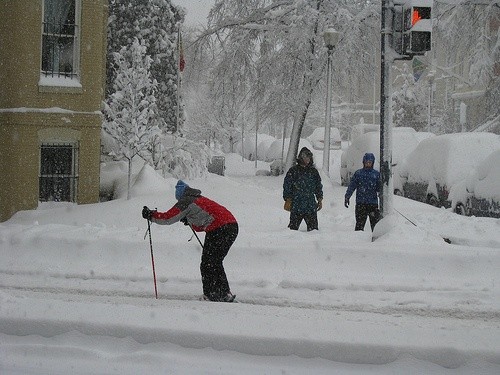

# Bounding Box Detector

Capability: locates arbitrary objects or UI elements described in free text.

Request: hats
[362,153,374,165]
[175,180,188,199]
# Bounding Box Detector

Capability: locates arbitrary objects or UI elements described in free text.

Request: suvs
[341,127,500,218]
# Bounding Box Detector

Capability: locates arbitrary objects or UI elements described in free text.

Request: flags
[177,32,185,71]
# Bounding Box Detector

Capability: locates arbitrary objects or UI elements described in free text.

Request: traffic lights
[391,0,432,55]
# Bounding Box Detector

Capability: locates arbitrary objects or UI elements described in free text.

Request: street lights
[321,23,344,176]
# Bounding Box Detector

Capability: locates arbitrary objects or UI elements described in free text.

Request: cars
[348,123,380,141]
[239,134,312,161]
[309,127,342,149]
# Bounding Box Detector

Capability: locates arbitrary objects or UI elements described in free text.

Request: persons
[142,180,239,302]
[344,153,383,231]
[283,147,323,232]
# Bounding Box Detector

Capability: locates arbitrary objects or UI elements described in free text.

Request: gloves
[317,201,322,211]
[344,198,349,208]
[142,206,152,221]
[283,199,292,211]
